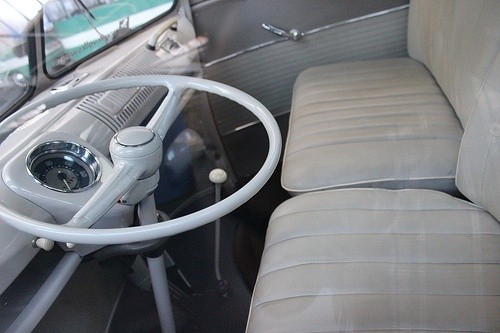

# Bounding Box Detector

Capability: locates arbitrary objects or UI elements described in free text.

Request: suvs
[0,34,71,89]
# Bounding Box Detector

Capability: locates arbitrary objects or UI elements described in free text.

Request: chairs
[281,0,500,199]
[243,53,500,333]
